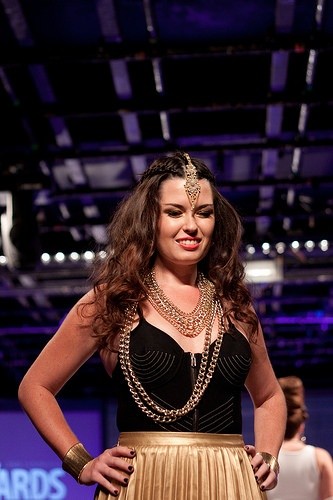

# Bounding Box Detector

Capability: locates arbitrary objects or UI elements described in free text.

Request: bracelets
[253,452,280,491]
[63,442,95,485]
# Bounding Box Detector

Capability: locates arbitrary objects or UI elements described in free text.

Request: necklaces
[119,269,224,422]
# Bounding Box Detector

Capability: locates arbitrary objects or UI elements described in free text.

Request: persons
[17,155,288,500]
[264,377,332,500]
